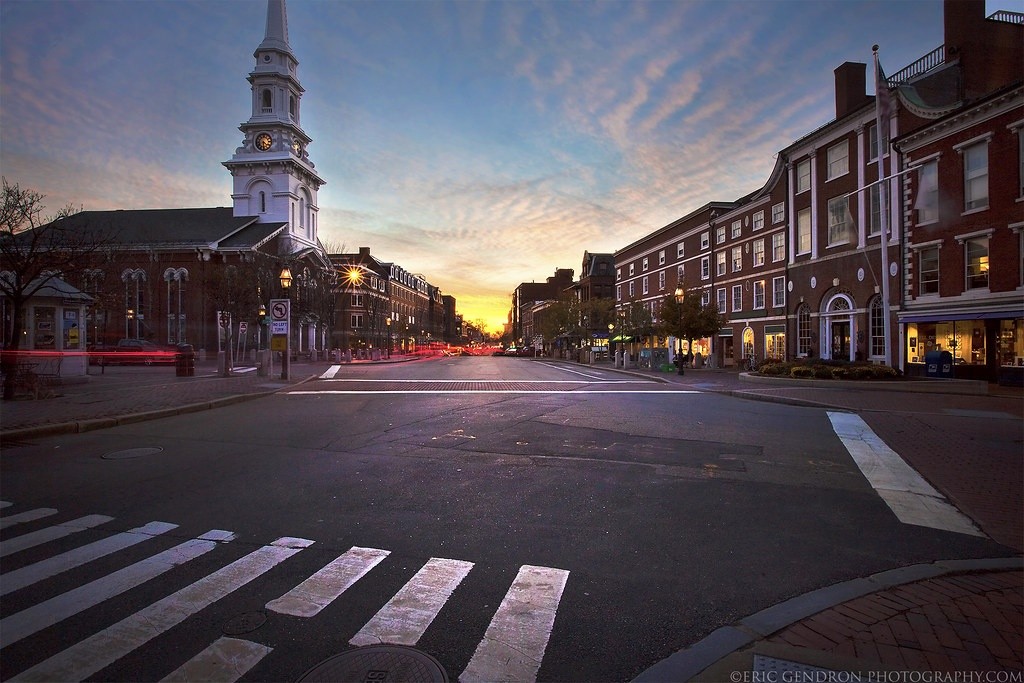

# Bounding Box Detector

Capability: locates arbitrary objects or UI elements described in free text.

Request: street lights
[385,317,392,359]
[256,303,267,369]
[674,281,686,377]
[618,310,626,358]
[279,266,293,381]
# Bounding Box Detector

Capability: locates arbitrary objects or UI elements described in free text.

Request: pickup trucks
[86,338,163,367]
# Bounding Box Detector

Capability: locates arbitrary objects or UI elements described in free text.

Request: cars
[504,345,539,357]
